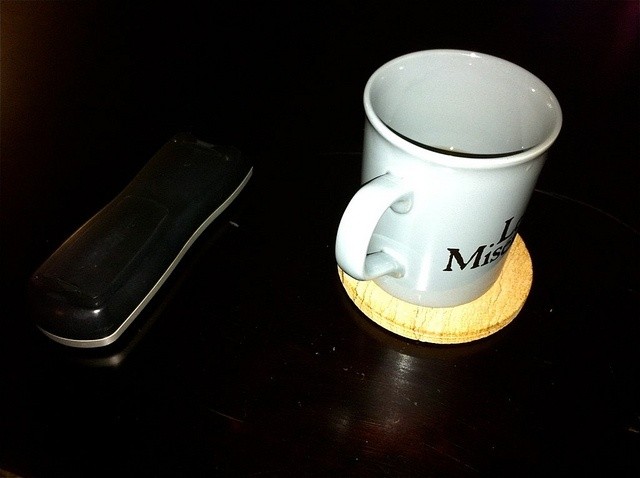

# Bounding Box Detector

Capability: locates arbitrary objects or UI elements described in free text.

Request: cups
[333,46,565,309]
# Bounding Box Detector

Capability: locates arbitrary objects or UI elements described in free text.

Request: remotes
[26,131,253,352]
